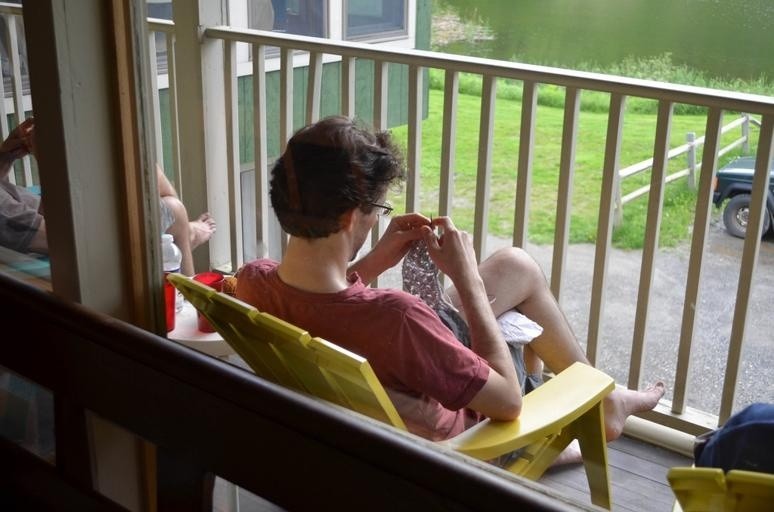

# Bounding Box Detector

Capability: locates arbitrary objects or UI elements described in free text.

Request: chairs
[165,269,615,511]
[668,468,772,511]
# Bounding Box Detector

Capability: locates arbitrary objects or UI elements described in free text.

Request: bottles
[160,234,183,313]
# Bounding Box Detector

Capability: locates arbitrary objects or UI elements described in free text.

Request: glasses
[372,200,393,216]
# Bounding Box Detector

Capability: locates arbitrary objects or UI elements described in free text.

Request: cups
[162,272,176,334]
[190,273,225,335]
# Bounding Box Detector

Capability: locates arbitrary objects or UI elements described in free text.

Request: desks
[165,298,236,359]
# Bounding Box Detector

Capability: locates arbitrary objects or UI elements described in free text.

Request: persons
[0,115,217,280]
[236,115,665,469]
[692,401,774,477]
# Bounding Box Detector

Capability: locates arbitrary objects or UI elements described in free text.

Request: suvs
[714,155,774,242]
